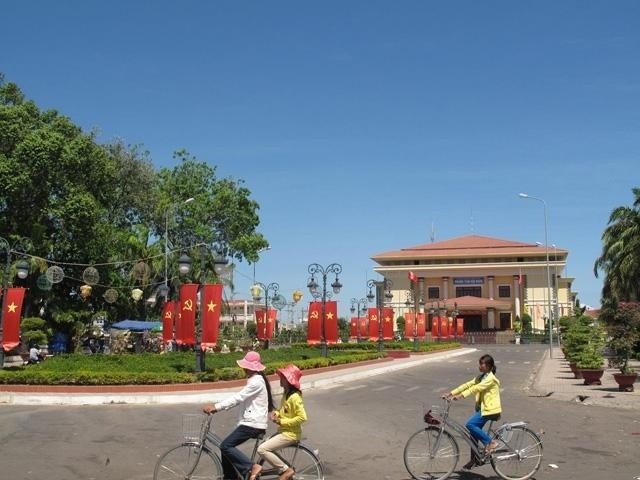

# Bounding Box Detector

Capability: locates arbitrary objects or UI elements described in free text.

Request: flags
[0,287,27,351]
[307,302,337,346]
[368,308,393,342]
[351,318,368,339]
[255,310,276,339]
[163,283,222,352]
[408,271,417,282]
[405,313,425,337]
[432,316,463,338]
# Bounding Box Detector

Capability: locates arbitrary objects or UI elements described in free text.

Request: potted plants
[561,315,605,385]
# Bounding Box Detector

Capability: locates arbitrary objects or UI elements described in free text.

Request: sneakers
[249,464,262,480]
[277,468,295,480]
[485,440,499,453]
[463,460,478,470]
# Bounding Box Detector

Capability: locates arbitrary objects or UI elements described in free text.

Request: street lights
[450,302,462,337]
[350,297,366,344]
[430,298,447,335]
[535,241,560,345]
[366,279,394,350]
[307,263,342,356]
[251,282,281,348]
[178,229,229,370]
[517,193,553,358]
[1,237,30,366]
[164,198,194,350]
[404,288,426,337]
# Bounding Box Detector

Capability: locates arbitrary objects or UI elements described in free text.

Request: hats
[276,363,303,389]
[236,351,266,371]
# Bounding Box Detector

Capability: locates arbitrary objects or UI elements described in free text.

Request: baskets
[422,402,442,425]
[181,414,204,439]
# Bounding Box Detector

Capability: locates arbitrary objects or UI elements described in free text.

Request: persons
[255,364,309,479]
[29,344,42,363]
[443,353,502,469]
[202,352,278,479]
[123,333,173,354]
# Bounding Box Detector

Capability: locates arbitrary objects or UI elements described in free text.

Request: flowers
[608,302,640,374]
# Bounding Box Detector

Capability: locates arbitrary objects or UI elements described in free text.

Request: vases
[612,373,637,391]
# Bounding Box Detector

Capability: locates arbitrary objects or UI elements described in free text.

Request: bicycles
[153,410,325,479]
[404,395,543,480]
